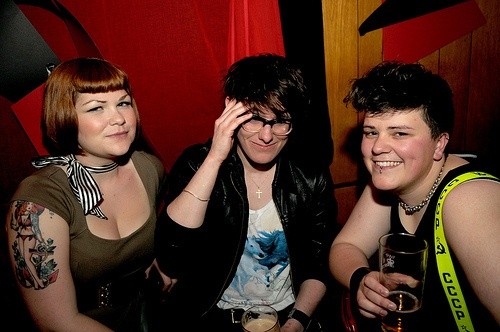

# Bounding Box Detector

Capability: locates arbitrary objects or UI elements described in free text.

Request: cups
[241,305,280,332]
[378,233,429,332]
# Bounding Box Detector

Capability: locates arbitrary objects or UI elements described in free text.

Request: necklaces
[236,146,273,199]
[396,160,448,216]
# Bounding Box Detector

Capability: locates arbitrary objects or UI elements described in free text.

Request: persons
[328,58,500,332]
[6,57,166,332]
[155,53,338,332]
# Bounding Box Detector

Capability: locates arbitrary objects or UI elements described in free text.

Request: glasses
[238,112,293,136]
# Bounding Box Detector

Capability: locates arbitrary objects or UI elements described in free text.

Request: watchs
[287,307,310,328]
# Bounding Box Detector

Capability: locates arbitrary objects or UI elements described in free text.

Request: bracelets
[181,188,211,203]
[345,267,373,294]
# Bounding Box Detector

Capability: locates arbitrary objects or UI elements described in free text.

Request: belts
[213,310,243,324]
